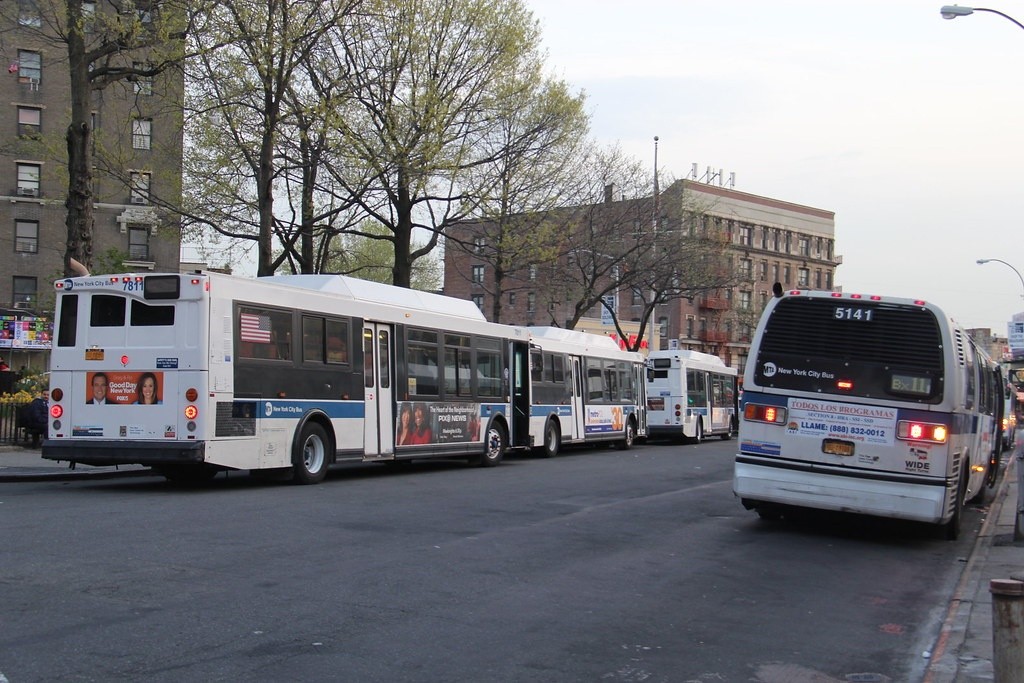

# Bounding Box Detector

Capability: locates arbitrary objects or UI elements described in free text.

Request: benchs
[13,406,49,449]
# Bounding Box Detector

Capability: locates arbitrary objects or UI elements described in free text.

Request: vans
[732,282,1015,542]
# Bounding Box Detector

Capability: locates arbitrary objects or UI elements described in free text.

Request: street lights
[940,5,1024,29]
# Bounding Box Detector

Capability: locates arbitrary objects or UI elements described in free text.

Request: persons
[133,372,163,405]
[33,389,49,439]
[86,372,116,405]
[397,402,412,445]
[467,412,480,441]
[412,403,432,444]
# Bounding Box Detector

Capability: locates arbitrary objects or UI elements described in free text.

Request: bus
[643,350,739,443]
[525,326,655,459]
[41,271,544,485]
